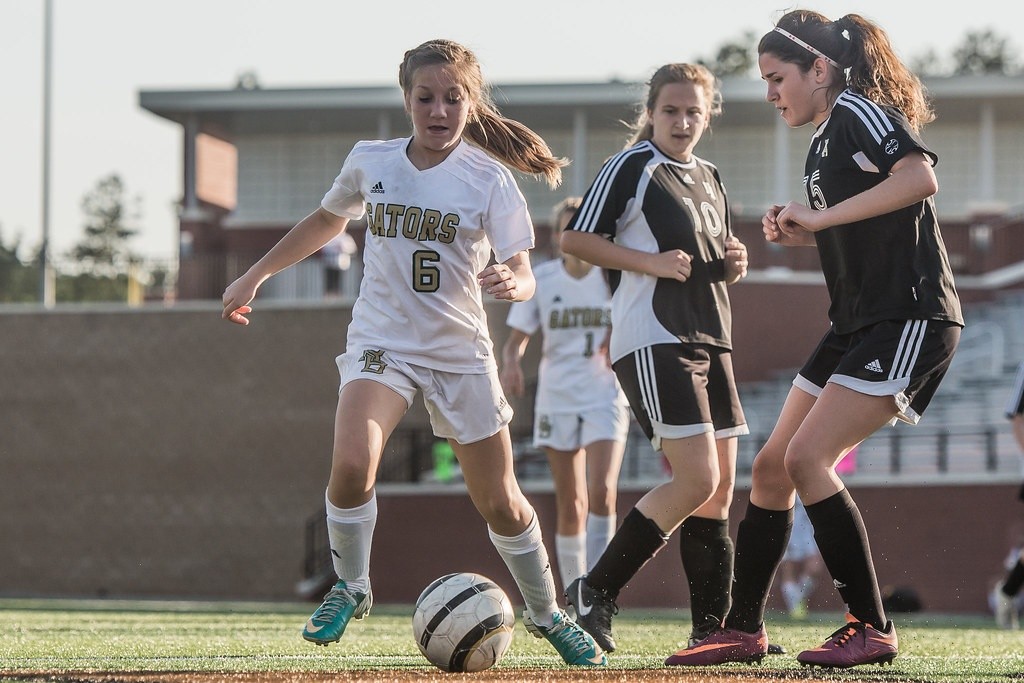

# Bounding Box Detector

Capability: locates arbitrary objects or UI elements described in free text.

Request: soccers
[410,570,514,671]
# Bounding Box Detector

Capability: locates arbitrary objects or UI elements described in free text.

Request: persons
[778,491,824,624]
[497,196,630,622]
[564,63,789,655]
[220,39,608,667]
[988,358,1024,632]
[665,8,964,668]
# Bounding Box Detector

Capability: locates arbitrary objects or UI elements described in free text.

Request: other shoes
[993,582,1020,630]
[790,599,808,619]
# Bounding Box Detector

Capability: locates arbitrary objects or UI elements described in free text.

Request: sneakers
[562,574,616,654]
[664,621,768,666]
[687,626,788,655]
[796,612,898,669]
[303,578,373,646]
[532,607,608,666]
